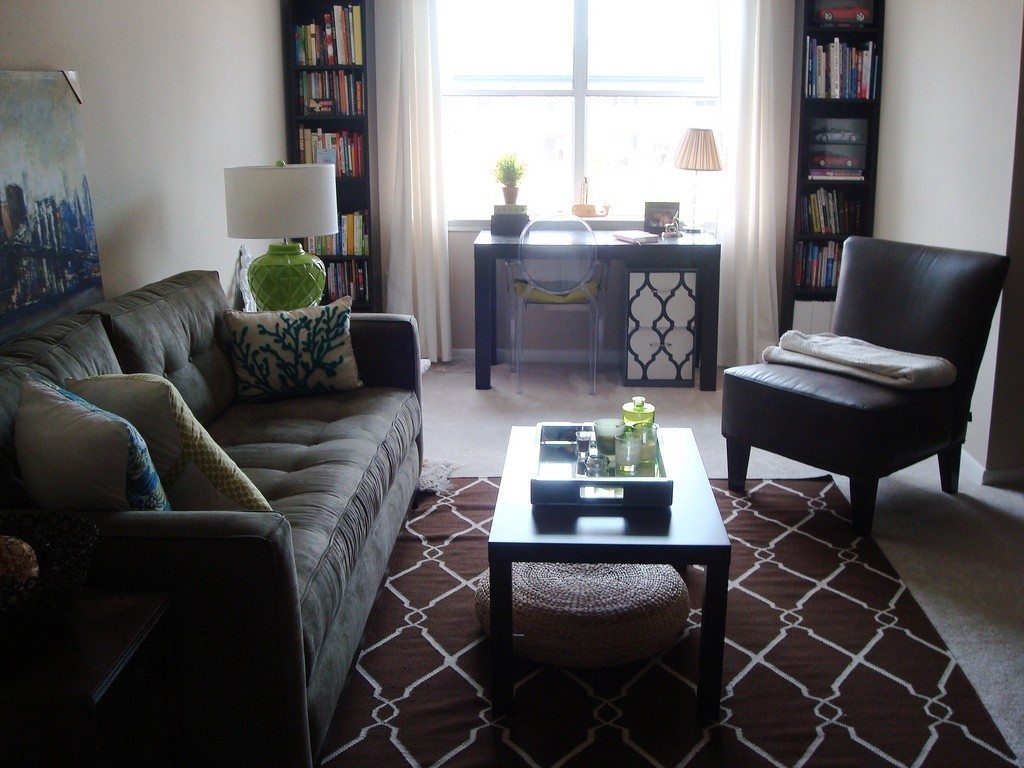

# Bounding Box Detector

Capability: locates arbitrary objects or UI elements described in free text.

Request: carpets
[313,470,1024,768]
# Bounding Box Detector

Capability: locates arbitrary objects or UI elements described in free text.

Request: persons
[650,210,673,227]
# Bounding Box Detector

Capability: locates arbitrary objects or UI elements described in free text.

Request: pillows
[64,372,273,513]
[7,376,170,512]
[224,294,365,408]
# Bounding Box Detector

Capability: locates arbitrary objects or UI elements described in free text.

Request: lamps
[224,159,339,310]
[671,128,724,234]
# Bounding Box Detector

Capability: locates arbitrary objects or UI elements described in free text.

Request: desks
[473,229,722,392]
[0,585,181,768]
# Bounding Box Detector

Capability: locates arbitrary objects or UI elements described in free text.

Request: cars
[815,152,861,169]
[815,6,872,22]
[811,127,860,142]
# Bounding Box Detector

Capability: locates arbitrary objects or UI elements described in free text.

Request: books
[303,209,369,256]
[800,187,861,234]
[807,169,865,180]
[294,4,364,66]
[298,123,365,179]
[796,241,842,287]
[317,258,368,302]
[298,71,366,115]
[805,35,878,100]
[613,230,658,243]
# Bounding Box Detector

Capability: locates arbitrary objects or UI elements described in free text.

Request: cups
[594,417,626,462]
[574,430,597,462]
[614,432,643,477]
[631,422,659,465]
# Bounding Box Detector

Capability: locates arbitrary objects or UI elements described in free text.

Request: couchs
[0,269,424,768]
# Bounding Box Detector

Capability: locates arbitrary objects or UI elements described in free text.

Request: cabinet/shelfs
[279,0,392,314]
[776,0,886,342]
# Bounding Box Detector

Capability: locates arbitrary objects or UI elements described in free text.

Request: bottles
[622,395,655,432]
[584,455,610,477]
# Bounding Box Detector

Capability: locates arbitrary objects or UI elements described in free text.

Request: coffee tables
[488,426,733,724]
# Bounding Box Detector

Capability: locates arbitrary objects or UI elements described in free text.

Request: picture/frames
[643,202,679,234]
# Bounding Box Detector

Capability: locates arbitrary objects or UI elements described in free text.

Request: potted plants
[494,154,527,205]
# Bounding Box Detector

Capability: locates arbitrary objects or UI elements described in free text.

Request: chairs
[504,208,609,396]
[721,236,1010,536]
